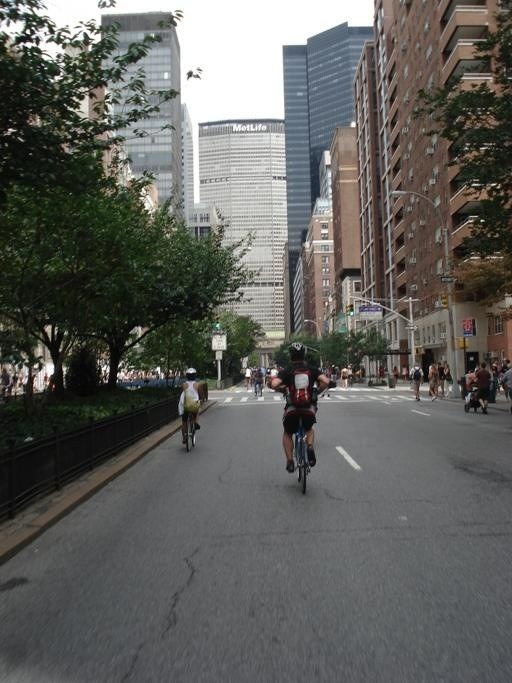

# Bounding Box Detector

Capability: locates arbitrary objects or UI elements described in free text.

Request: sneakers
[192,422,200,430]
[183,437,186,443]
[482,407,487,414]
[307,447,316,467]
[286,463,294,472]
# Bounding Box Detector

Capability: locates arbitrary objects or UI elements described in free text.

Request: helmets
[288,343,305,352]
[186,368,196,374]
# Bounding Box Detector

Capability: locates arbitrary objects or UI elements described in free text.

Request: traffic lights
[216,324,220,330]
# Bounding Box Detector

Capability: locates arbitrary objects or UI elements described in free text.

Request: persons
[408,360,424,400]
[378,366,385,381]
[268,341,331,471]
[426,357,511,413]
[0,363,56,398]
[401,364,408,382]
[242,362,365,396]
[85,362,180,385]
[392,366,399,383]
[181,366,203,443]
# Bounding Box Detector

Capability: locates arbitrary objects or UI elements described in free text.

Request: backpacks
[414,367,420,381]
[289,367,314,407]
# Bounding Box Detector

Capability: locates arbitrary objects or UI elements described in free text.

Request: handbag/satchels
[184,397,200,414]
[446,376,452,380]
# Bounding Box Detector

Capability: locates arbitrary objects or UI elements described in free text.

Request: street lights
[303,319,323,373]
[349,294,419,367]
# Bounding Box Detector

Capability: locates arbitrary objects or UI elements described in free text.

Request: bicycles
[246,377,262,396]
[184,399,203,451]
[349,375,353,387]
[294,418,312,495]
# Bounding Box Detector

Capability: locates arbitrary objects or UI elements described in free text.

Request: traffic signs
[358,306,383,322]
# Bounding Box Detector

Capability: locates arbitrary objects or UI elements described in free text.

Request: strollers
[465,382,481,412]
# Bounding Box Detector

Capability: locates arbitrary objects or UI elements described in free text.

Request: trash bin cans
[389,375,396,387]
[460,377,497,402]
